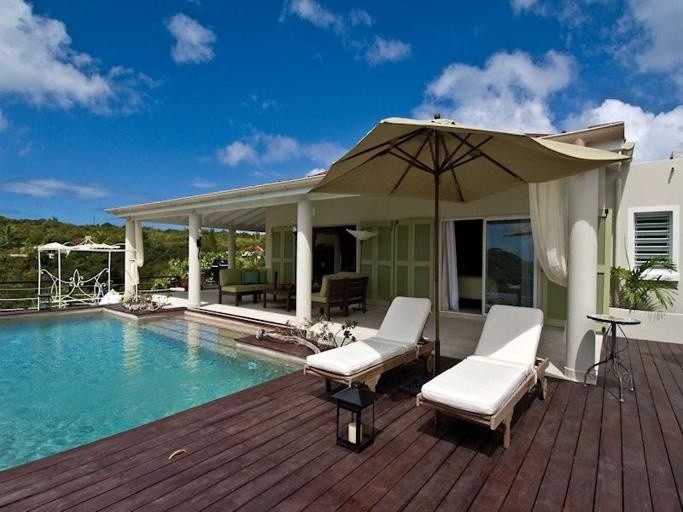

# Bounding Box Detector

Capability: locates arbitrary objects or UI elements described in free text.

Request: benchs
[219,268,278,306]
[312,273,370,322]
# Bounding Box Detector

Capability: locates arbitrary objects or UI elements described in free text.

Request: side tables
[581,312,641,404]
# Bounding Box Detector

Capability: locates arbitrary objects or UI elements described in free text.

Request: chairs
[416,304,551,450]
[303,297,436,396]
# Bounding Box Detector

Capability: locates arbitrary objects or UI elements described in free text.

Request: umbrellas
[305,110,632,377]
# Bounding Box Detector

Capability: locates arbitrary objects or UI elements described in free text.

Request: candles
[348,421,363,444]
